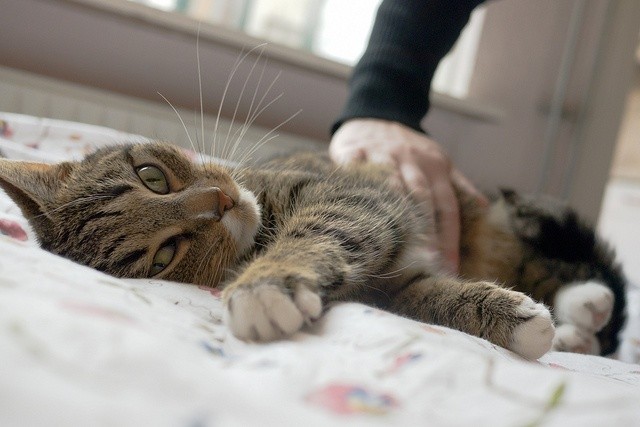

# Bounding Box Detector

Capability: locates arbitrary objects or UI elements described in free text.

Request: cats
[0,27,626,359]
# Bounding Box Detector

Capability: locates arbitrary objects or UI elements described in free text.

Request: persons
[326,0,488,276]
